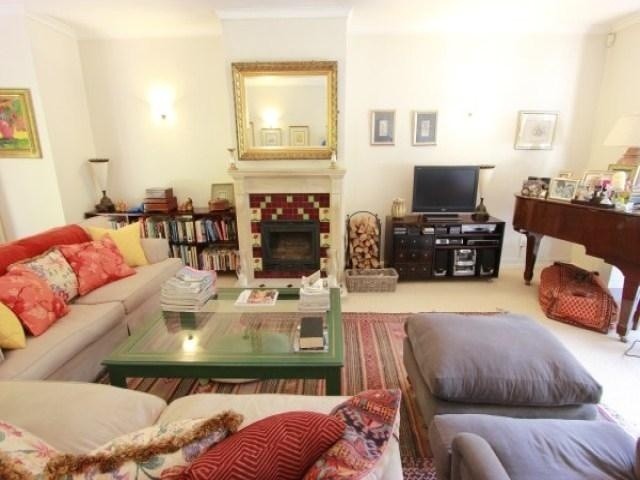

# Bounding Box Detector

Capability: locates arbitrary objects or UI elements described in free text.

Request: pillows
[405,312,602,406]
[0,220,151,350]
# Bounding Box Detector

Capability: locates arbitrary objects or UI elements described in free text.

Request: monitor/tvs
[411,165,480,221]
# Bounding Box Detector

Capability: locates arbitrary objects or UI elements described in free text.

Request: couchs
[0,216,184,382]
[0,379,405,480]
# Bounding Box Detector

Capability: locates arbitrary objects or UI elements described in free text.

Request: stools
[402,311,603,429]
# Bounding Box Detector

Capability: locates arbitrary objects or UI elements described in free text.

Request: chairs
[427,411,636,480]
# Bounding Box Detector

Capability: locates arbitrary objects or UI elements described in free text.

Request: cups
[595,191,607,198]
[117,199,128,212]
[624,202,633,212]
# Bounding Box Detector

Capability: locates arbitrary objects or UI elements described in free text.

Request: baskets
[346,268,399,293]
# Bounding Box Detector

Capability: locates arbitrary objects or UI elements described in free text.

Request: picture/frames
[413,111,437,145]
[514,110,559,151]
[249,122,310,146]
[370,109,396,145]
[0,88,43,159]
[521,147,640,202]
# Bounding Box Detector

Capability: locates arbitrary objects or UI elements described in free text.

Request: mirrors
[230,61,337,160]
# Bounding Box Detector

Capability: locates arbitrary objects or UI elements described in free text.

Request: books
[294,317,329,354]
[236,288,280,307]
[108,186,243,313]
[297,286,331,310]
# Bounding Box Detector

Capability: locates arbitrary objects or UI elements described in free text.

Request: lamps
[471,166,495,220]
[88,157,116,213]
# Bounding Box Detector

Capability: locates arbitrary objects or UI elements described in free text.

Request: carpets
[97,311,507,480]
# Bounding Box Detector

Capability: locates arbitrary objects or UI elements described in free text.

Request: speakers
[432,249,449,277]
[479,247,497,276]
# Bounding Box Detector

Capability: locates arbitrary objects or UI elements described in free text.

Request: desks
[102,289,345,394]
[513,193,640,342]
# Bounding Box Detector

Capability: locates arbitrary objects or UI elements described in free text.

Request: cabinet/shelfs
[384,214,506,281]
[84,206,240,278]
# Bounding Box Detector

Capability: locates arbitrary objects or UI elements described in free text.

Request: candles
[331,150,336,162]
[229,150,236,163]
